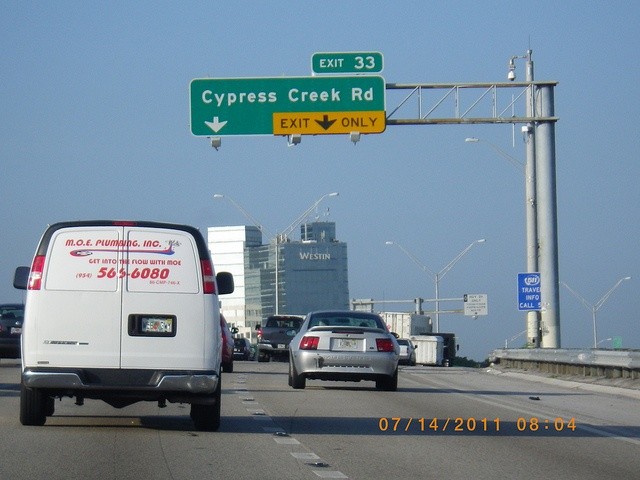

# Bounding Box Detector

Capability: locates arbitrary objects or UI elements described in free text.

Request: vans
[12,219,235,426]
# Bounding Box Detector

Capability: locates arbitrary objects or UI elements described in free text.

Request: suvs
[233,338,256,361]
[255,314,304,362]
[0,301,24,361]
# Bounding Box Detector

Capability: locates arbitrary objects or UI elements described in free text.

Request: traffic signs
[189,52,389,134]
[517,272,540,311]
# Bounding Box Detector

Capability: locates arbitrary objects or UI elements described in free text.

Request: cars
[220,313,235,374]
[287,311,401,390]
[396,337,417,366]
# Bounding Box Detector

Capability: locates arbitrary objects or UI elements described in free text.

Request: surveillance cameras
[509,70,516,83]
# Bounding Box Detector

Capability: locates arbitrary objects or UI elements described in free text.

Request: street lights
[558,276,631,348]
[384,239,486,333]
[464,137,535,346]
[214,192,340,314]
